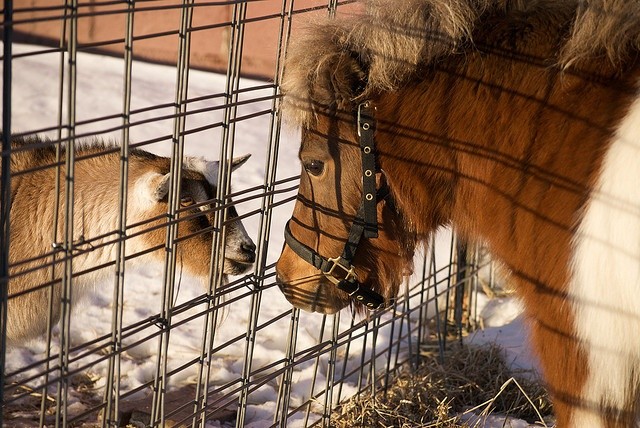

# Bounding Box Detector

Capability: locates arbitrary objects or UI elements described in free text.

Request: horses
[275,0,639,428]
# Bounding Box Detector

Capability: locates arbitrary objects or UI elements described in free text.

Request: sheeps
[0,133,258,352]
